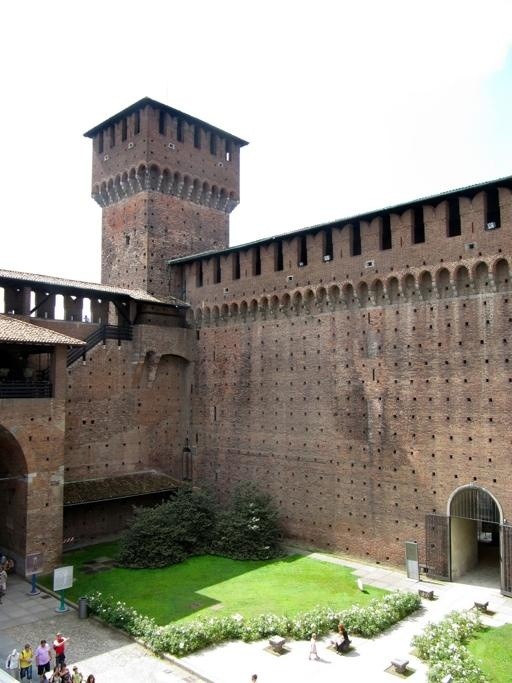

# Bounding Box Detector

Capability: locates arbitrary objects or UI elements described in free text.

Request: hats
[11,649,16,656]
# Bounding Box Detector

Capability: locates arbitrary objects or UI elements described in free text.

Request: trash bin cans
[79,597,88,619]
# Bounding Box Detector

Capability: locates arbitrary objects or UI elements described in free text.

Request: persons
[308,632,321,660]
[5,631,98,683]
[335,622,350,655]
[0,553,16,605]
[251,674,261,683]
